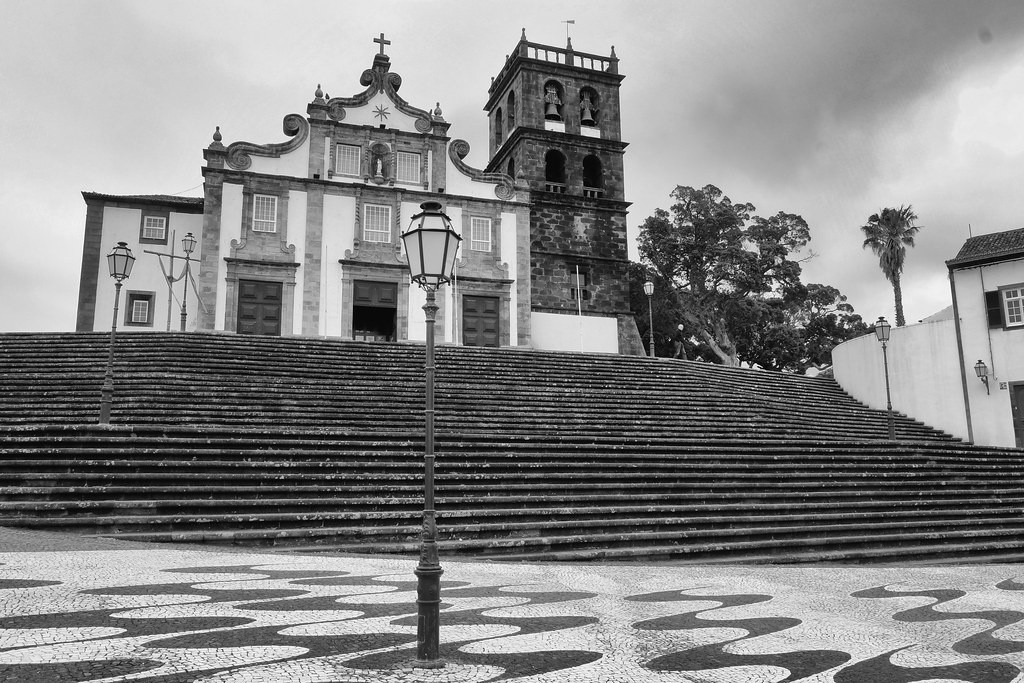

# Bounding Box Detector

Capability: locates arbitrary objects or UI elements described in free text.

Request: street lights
[644,279,655,358]
[181,231,197,331]
[874,315,896,440]
[98,241,135,424]
[399,194,465,670]
